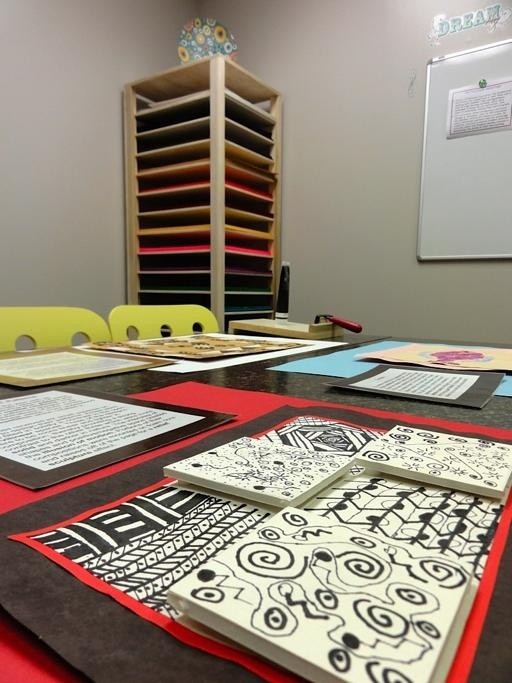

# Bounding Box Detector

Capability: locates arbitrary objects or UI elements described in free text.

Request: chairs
[107,303,220,340]
[0,306,111,353]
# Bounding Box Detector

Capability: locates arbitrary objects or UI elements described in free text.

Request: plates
[179,17,240,62]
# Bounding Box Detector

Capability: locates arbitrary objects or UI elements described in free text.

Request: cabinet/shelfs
[124,52,282,333]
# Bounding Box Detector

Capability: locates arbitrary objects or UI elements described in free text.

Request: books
[167,507,476,683]
[161,435,355,508]
[356,423,511,498]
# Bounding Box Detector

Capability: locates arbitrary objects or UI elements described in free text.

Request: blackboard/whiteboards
[416,38,512,261]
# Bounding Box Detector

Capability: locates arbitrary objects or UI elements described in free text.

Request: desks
[0,335,512,683]
